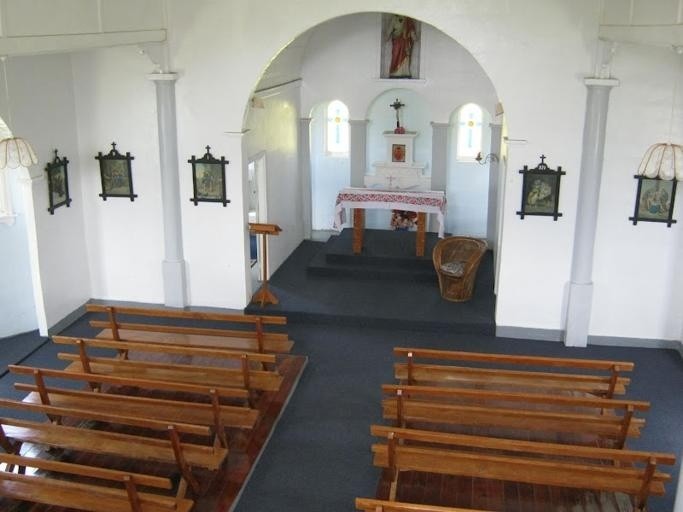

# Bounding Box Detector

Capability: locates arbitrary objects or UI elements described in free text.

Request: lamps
[0,54,38,170]
[638,45,683,183]
[475,152,499,165]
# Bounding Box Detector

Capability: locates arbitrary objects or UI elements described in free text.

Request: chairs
[432,236,487,302]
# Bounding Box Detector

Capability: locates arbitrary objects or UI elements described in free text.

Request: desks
[332,186,447,257]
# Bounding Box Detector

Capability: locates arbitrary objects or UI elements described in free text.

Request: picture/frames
[45,149,72,214]
[628,175,677,227]
[187,145,230,207]
[95,142,138,201]
[516,155,566,222]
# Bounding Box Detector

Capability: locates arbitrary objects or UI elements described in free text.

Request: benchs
[0,305,294,512]
[356,347,676,512]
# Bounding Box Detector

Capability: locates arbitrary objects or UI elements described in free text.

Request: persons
[385,13,419,80]
